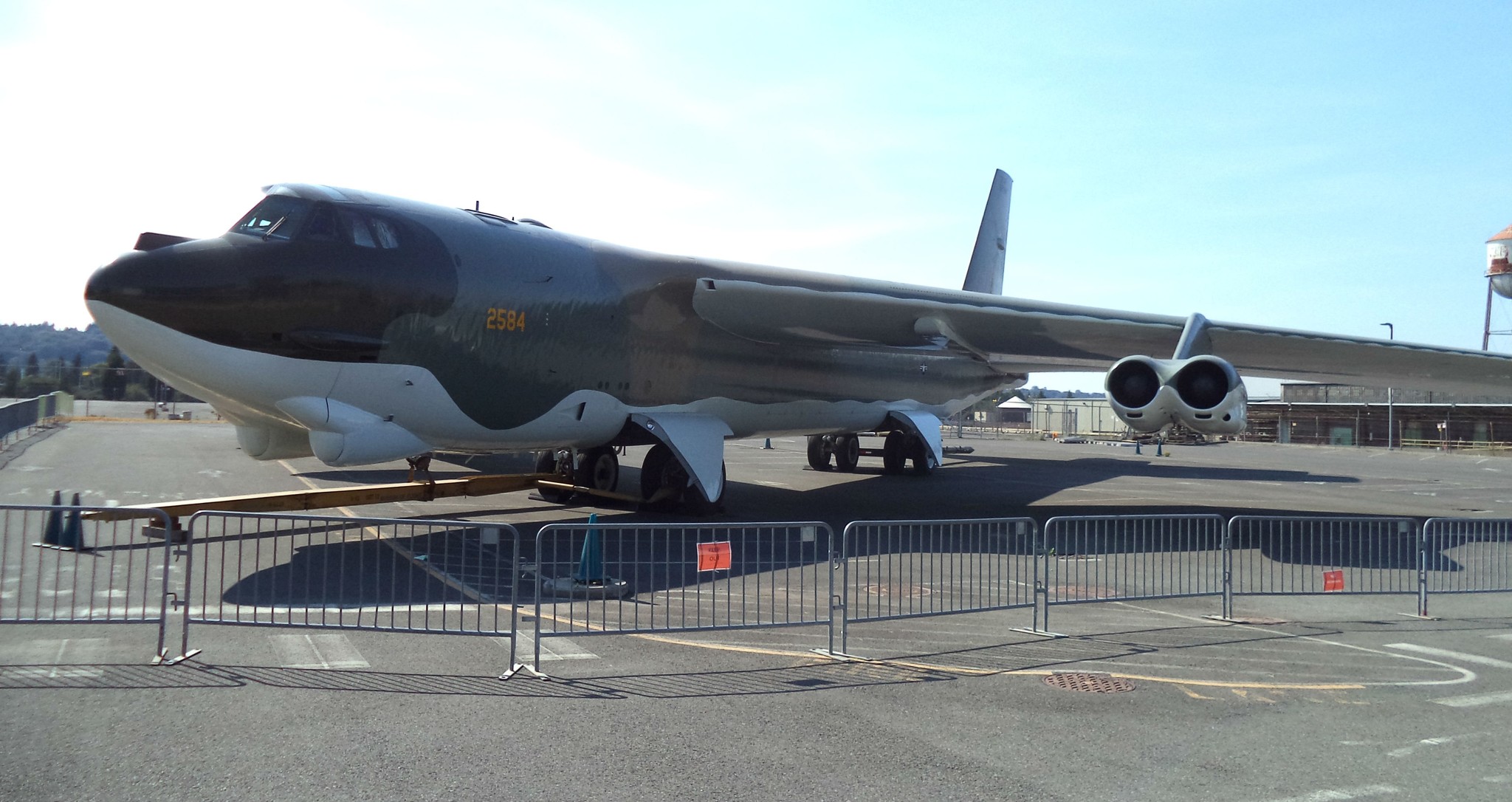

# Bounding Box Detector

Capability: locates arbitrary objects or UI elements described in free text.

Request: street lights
[1379,323,1393,340]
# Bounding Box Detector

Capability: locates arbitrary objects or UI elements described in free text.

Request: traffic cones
[759,437,775,449]
[1133,440,1143,455]
[572,513,612,582]
[32,489,64,547]
[51,491,94,551]
[1155,440,1164,456]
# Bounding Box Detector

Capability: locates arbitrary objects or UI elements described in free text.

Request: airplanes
[83,168,1511,523]
